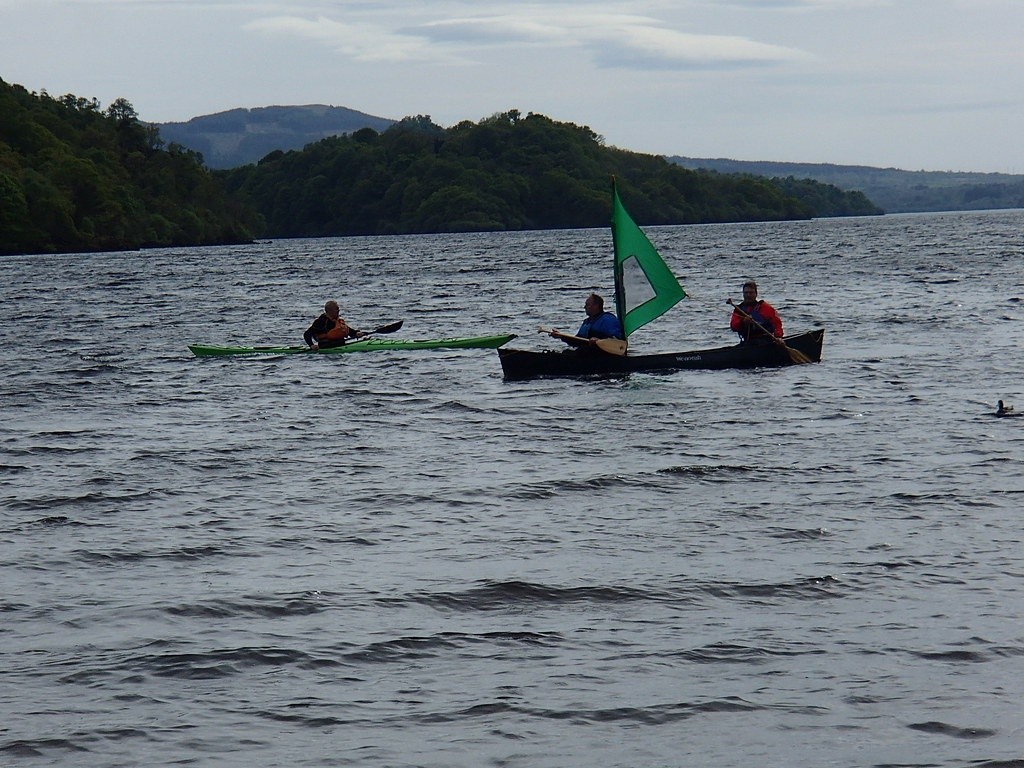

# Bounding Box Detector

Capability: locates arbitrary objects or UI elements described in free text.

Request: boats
[497,174,825,382]
[188,334,518,356]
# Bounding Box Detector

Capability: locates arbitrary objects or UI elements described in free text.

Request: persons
[548,294,625,356]
[303,301,364,351]
[730,283,785,345]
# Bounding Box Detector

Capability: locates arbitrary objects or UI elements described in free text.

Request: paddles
[536,325,627,357]
[726,297,811,364]
[317,320,406,348]
